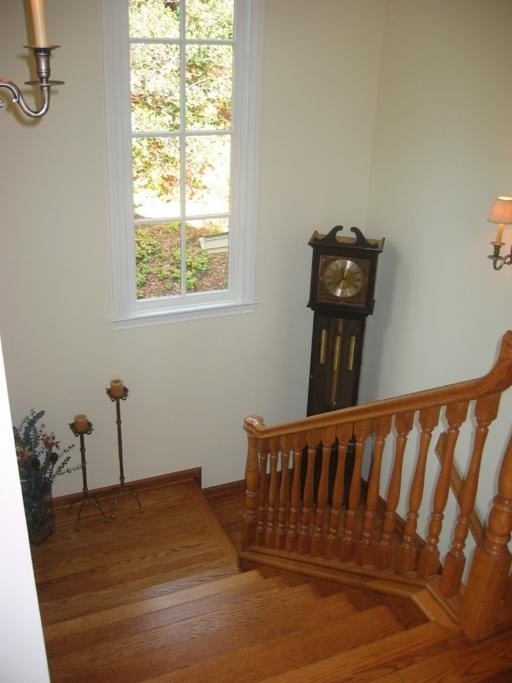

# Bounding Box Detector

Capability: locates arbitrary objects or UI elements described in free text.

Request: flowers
[13,411,86,542]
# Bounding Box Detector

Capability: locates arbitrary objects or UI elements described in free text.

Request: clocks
[294,225,385,506]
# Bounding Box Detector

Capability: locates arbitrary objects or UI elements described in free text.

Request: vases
[23,482,55,545]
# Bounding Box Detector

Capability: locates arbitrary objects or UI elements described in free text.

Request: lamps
[487,196,512,270]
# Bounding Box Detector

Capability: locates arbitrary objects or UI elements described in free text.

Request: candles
[74,415,88,431]
[28,1,50,47]
[110,379,124,396]
